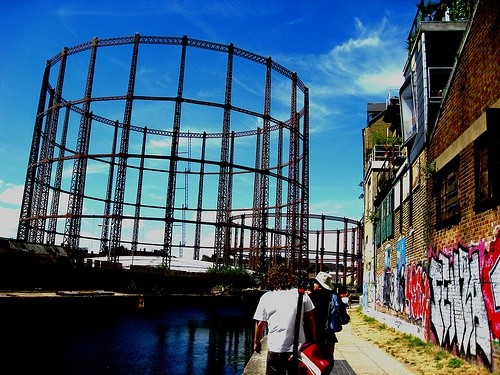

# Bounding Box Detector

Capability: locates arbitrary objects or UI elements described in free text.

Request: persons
[305,271,351,375]
[252,263,316,375]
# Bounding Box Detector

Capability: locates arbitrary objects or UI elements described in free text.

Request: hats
[310,271,334,290]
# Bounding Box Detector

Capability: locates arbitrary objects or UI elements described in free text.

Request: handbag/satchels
[287,342,334,375]
[325,293,343,332]
